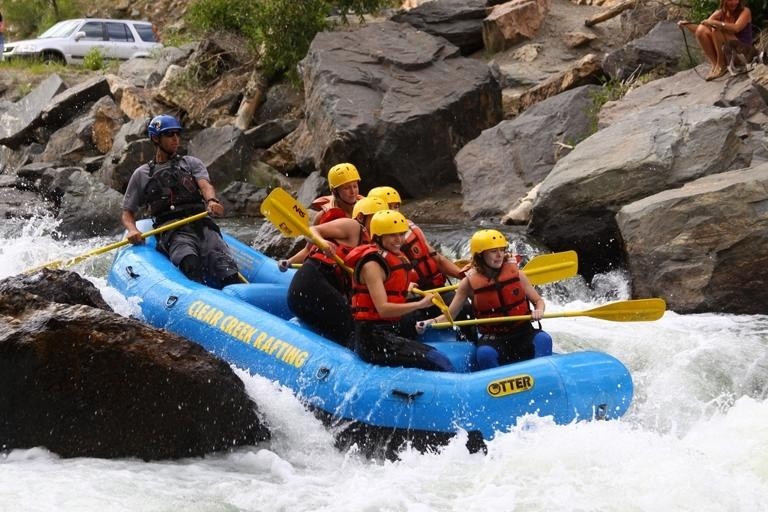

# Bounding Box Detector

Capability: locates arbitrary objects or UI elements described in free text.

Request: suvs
[3,19,164,66]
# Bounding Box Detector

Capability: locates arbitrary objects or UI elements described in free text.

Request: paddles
[411,287,454,326]
[260,187,355,275]
[423,250,578,294]
[419,298,666,329]
[25,210,208,273]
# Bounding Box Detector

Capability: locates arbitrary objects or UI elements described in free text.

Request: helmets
[469,229,509,258]
[369,209,409,251]
[366,186,402,204]
[351,197,390,220]
[328,162,361,206]
[147,114,183,156]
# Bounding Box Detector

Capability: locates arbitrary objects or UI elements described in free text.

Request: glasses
[161,130,182,138]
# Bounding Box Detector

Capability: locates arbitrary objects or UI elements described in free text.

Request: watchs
[721,22,725,30]
[205,197,220,206]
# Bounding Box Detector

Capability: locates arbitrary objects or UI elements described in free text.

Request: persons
[120,117,241,288]
[366,187,472,291]
[304,163,366,226]
[678,1,753,82]
[287,195,388,350]
[344,210,454,378]
[415,230,554,369]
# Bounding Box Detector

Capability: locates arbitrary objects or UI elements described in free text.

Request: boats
[107,218,634,463]
